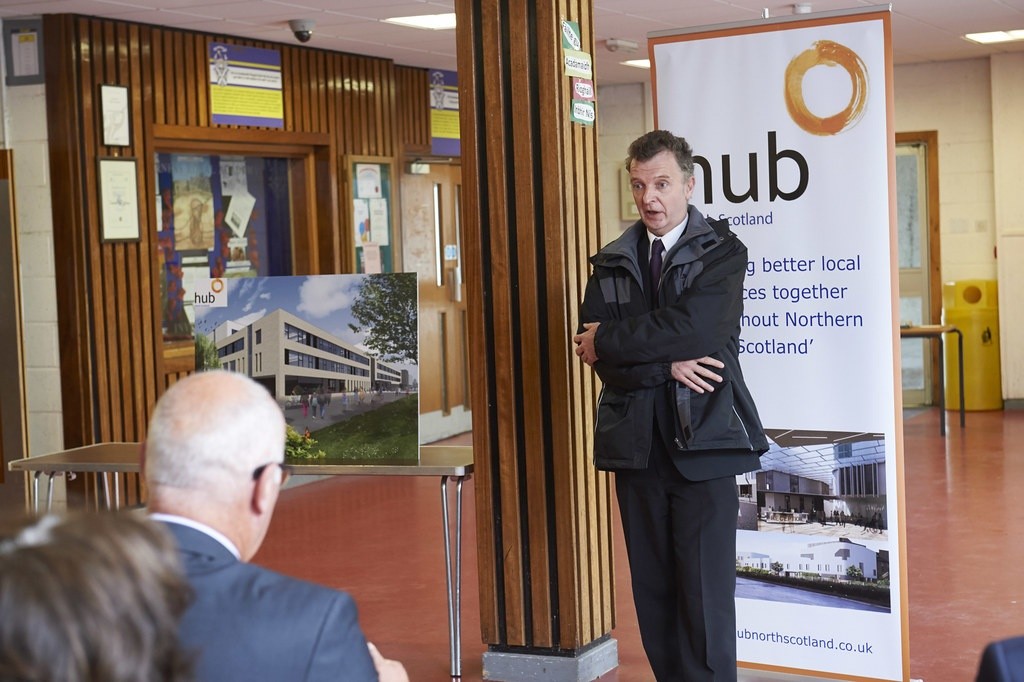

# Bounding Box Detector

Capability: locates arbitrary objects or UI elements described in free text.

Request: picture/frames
[98,157,140,242]
[98,84,133,147]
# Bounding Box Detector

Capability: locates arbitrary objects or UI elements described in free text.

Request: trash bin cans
[942,278,1004,412]
[976,634,1024,682]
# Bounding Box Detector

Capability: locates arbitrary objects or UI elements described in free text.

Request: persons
[574,130,759,682]
[0,510,198,682]
[143,370,409,682]
[768,506,884,534]
[300,384,408,420]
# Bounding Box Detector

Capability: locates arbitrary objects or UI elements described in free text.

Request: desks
[900,325,965,438]
[7,441,474,682]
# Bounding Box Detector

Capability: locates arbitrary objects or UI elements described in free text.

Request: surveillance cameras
[288,18,319,42]
